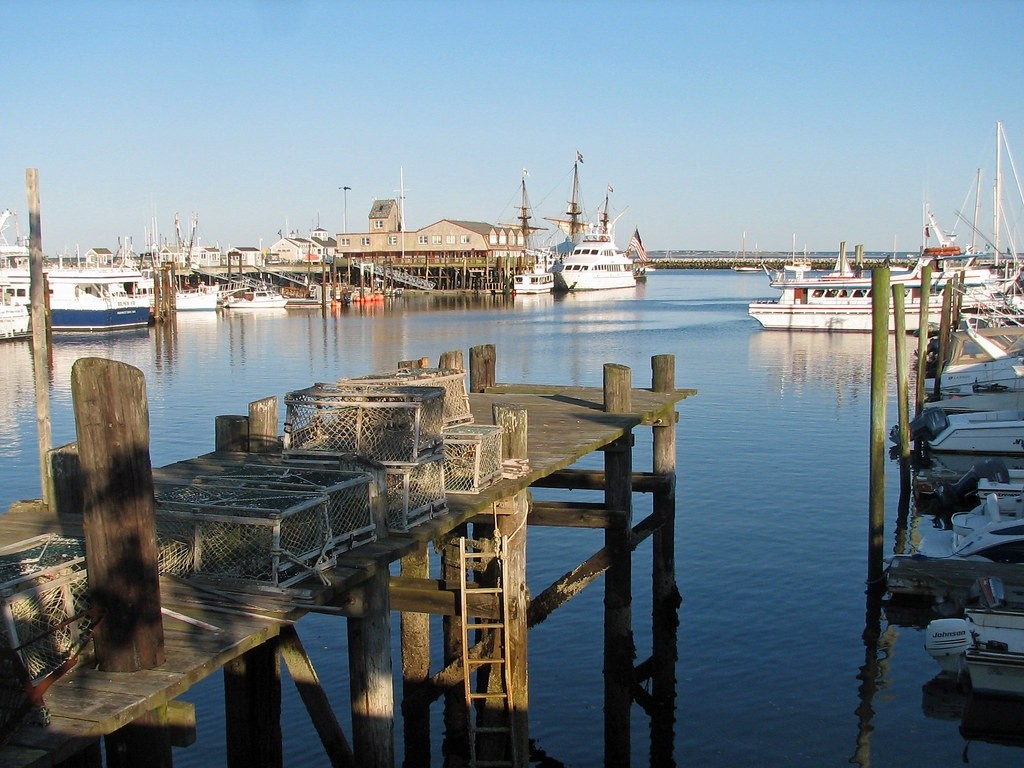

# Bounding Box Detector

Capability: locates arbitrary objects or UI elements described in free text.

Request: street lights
[338,186,352,234]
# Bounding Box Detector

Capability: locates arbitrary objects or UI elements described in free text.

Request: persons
[855,262,862,278]
[882,255,891,268]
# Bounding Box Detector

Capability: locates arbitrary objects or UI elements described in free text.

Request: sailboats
[483,149,657,279]
[731,231,763,274]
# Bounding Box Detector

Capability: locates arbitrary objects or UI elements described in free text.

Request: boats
[923,575,1024,697]
[746,121,1024,462]
[918,492,1024,564]
[948,455,1024,506]
[508,271,556,295]
[0,203,409,340]
[918,668,1024,764]
[554,205,638,291]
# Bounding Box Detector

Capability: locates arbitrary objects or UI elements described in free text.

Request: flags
[631,229,647,263]
